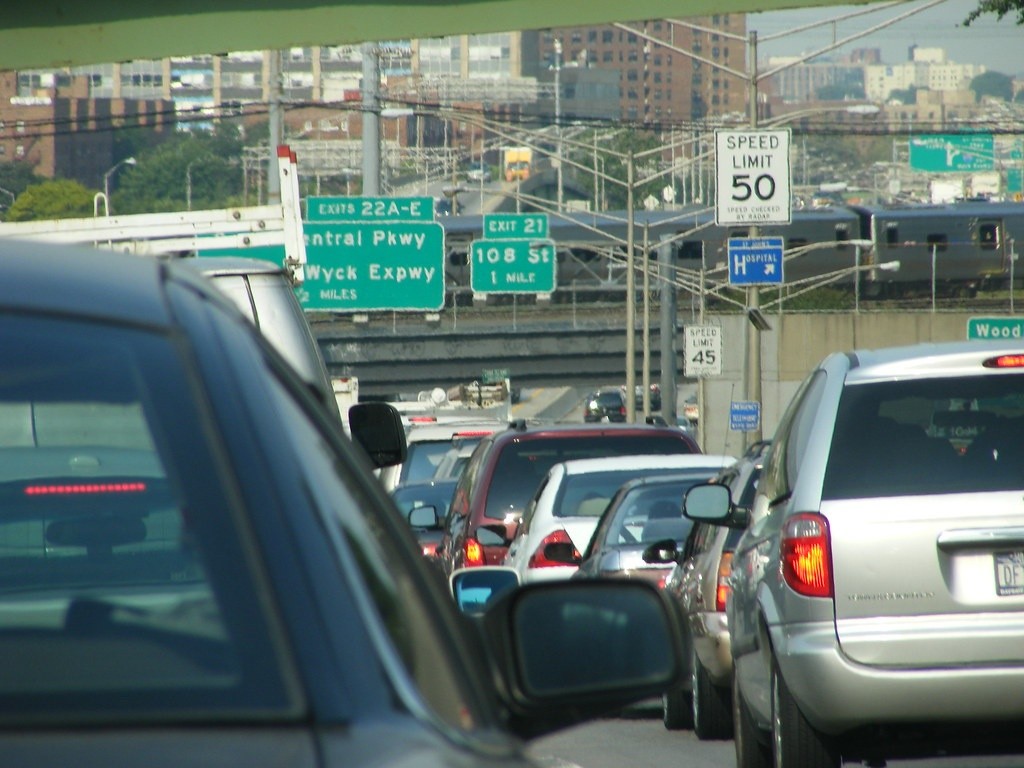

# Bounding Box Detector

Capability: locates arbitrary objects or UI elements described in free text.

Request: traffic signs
[909,134,994,173]
[728,236,784,287]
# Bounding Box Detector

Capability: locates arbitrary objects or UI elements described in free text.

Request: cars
[436,200,464,214]
[584,385,628,423]
[465,163,493,183]
[630,385,661,411]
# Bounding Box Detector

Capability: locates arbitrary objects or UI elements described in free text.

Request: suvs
[379,336,1024,768]
[0,237,695,768]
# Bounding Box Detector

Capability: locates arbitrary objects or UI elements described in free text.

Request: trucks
[504,148,534,181]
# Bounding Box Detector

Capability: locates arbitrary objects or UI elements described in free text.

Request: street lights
[555,39,563,211]
[105,157,136,251]
[441,181,846,425]
[379,103,879,425]
[528,237,900,453]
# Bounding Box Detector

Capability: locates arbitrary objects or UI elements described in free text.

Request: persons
[649,502,682,520]
[647,545,674,562]
